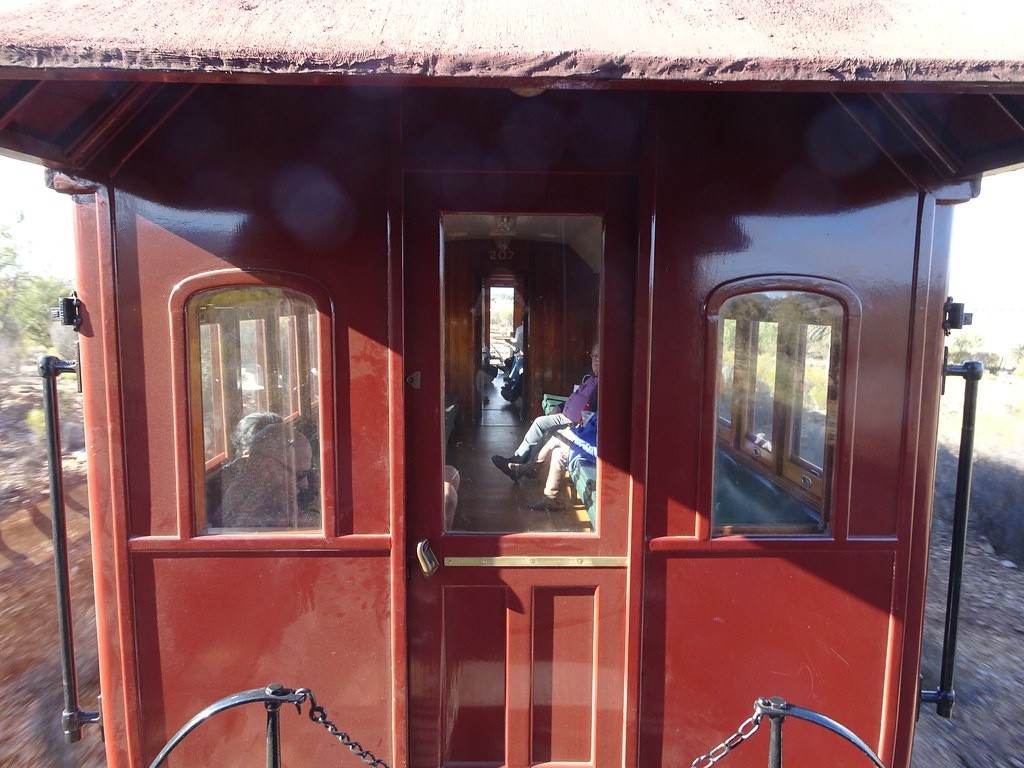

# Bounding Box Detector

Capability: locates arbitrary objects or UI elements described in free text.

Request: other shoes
[496,357,518,405]
[507,457,539,480]
[492,455,523,481]
[526,495,560,512]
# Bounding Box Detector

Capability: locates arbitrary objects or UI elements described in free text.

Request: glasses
[261,455,312,484]
[589,354,600,360]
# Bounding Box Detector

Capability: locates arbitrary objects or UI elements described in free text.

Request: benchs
[541,393,821,535]
[201,390,461,529]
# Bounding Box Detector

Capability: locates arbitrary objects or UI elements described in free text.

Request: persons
[207,423,321,530]
[230,411,285,480]
[508,410,598,512]
[492,343,599,481]
[482,318,524,403]
[443,465,460,532]
